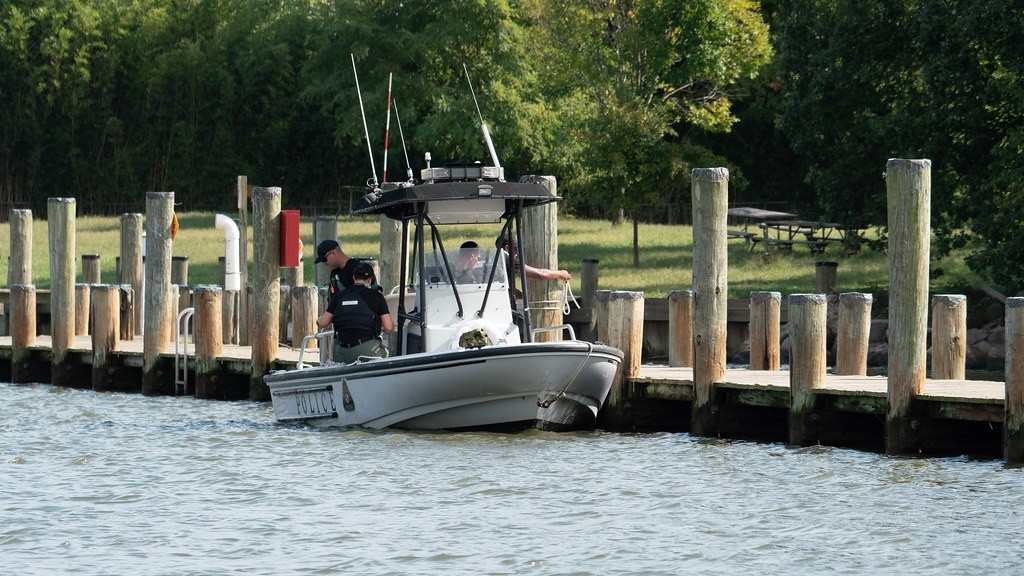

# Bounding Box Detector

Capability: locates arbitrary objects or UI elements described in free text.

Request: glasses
[322,252,331,262]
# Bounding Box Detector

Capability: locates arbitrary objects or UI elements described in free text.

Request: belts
[341,336,373,348]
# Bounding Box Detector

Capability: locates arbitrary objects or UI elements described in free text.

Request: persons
[434,234,569,298]
[313,240,384,360]
[315,264,395,364]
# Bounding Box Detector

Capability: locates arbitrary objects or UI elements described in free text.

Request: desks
[763,220,844,256]
[728,207,800,253]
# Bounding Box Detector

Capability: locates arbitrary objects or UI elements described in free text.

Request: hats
[352,263,374,277]
[314,240,338,263]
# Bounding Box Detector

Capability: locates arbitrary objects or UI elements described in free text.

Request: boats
[261,51,625,431]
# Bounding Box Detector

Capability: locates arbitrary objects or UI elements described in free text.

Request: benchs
[751,237,803,245]
[808,237,858,245]
[760,224,818,234]
[727,231,757,239]
